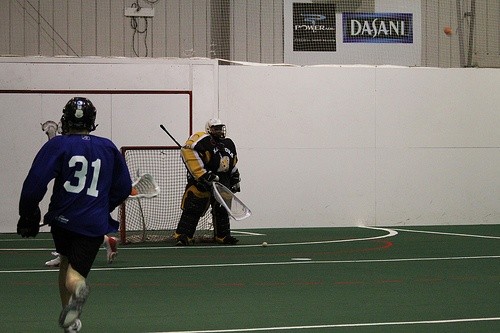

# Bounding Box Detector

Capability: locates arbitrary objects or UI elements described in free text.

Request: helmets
[205,118,226,138]
[62,97,97,129]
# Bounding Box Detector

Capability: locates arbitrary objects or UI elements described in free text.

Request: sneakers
[174,235,189,246]
[214,235,236,245]
[59,281,90,333]
[45,256,61,266]
[106,237,118,263]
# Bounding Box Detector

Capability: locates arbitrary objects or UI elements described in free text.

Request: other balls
[262,242,267,246]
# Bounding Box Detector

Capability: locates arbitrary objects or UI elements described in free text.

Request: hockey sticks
[38,172,160,227]
[41,120,59,140]
[160,124,253,221]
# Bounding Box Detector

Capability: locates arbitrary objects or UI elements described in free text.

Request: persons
[175,117,241,246]
[17,97,133,333]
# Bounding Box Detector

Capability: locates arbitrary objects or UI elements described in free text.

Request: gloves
[16,213,41,238]
[203,170,219,187]
[229,173,240,193]
